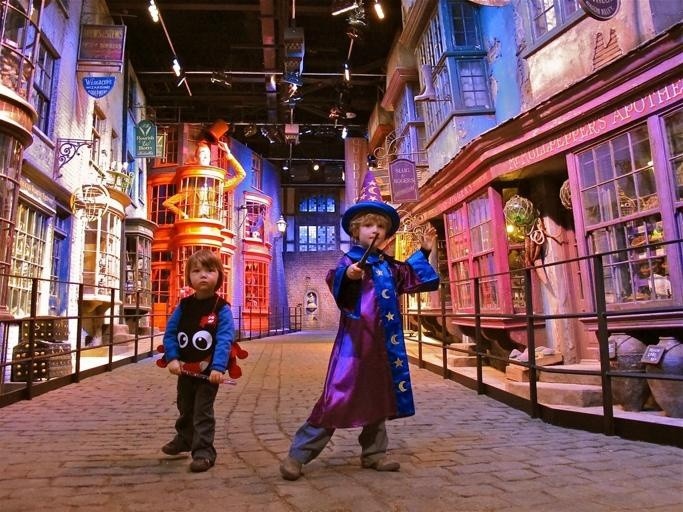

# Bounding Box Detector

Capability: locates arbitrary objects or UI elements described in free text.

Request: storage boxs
[9,317,79,382]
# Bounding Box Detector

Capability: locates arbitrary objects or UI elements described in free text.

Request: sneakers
[280,455,302,481]
[361,457,400,470]
[161,441,183,455]
[189,457,212,471]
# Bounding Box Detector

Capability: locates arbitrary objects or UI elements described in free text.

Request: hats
[342,171,400,236]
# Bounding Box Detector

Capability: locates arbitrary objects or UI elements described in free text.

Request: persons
[161,138,247,222]
[278,208,439,483]
[157,249,235,474]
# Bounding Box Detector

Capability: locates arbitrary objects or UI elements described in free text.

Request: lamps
[274,214,287,244]
